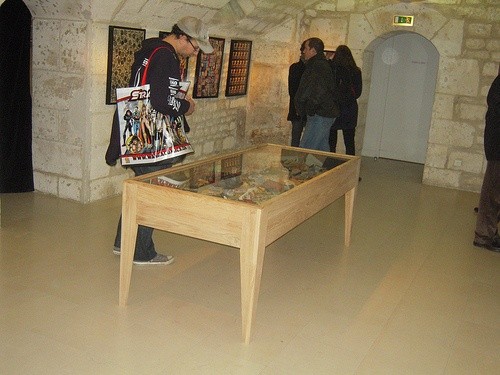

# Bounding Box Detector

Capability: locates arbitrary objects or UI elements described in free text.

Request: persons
[104,16,216,268]
[472,66,500,253]
[292,37,340,155]
[286,40,310,147]
[326,44,363,183]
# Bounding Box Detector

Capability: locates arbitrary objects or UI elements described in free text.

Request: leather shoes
[473,238,500,252]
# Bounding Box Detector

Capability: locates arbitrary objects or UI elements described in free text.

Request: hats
[176,16,214,54]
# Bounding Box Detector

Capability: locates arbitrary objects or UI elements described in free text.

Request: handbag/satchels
[116,47,195,166]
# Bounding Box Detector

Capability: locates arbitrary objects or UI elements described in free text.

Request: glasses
[187,35,198,51]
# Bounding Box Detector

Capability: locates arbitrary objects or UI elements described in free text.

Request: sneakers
[113,246,121,255]
[133,253,174,265]
